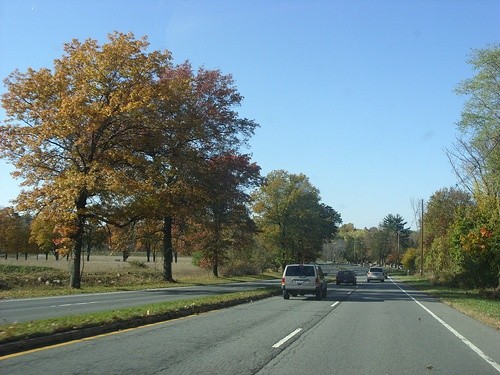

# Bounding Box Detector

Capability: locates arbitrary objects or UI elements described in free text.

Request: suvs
[281,264,328,298]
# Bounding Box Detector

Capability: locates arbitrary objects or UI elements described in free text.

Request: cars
[367,268,384,284]
[335,270,358,285]
[383,268,387,279]
